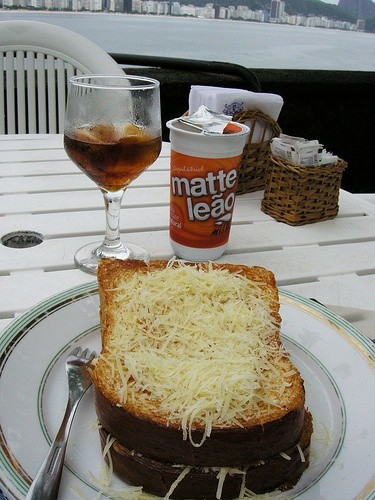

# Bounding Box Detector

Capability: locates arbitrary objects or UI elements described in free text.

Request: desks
[0,135,375,344]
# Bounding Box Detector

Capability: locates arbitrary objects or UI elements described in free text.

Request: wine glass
[64,75,162,274]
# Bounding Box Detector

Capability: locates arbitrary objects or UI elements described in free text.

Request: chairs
[0,20,133,135]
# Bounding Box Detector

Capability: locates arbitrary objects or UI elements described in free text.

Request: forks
[25,346,100,500]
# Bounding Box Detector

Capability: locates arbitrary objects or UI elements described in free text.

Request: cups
[166,118,251,262]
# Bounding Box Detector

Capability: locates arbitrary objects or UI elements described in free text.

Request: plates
[1,278,374,500]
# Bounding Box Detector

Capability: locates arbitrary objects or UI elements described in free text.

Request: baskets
[179,109,282,195]
[260,152,348,227]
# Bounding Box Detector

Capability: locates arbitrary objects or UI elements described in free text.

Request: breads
[92,258,312,500]
